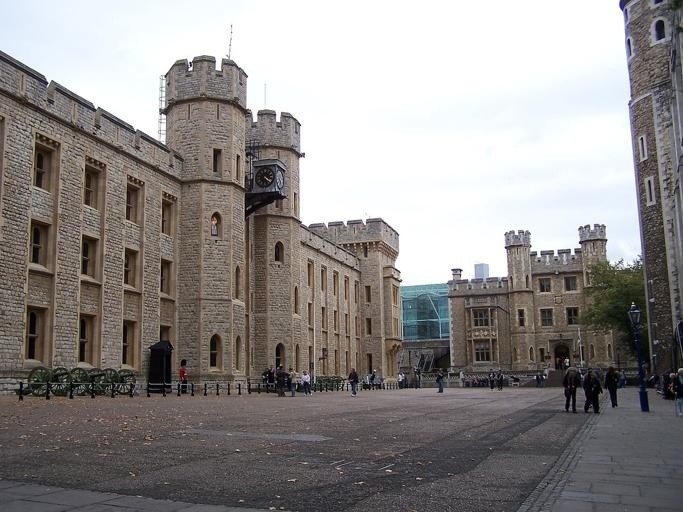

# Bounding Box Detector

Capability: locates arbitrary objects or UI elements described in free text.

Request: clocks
[275,170,284,189]
[254,167,274,187]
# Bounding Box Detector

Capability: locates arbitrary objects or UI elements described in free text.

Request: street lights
[626,299,649,413]
[615,347,620,371]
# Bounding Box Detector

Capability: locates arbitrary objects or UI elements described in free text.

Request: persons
[347,367,359,398]
[532,355,682,424]
[178,358,188,394]
[360,367,505,394]
[260,363,314,399]
